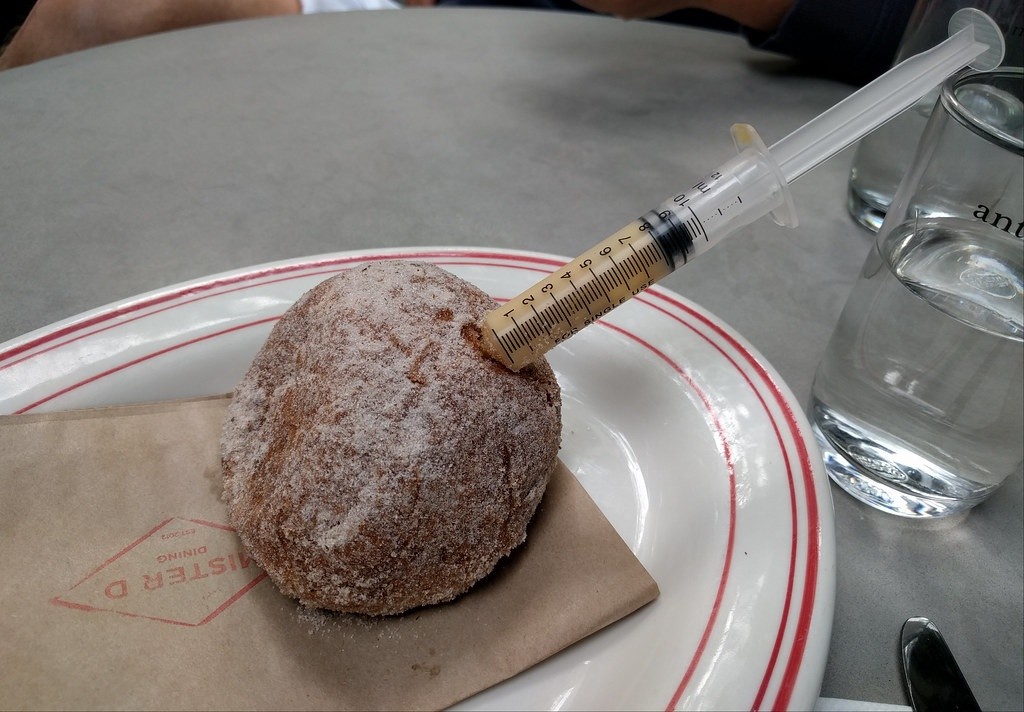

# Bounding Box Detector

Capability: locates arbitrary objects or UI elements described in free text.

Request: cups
[846,0,1024,233]
[806,67,1024,518]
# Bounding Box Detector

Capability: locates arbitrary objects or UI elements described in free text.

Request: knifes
[900,616,982,712]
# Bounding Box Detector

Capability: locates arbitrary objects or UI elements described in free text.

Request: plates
[0,244,835,712]
